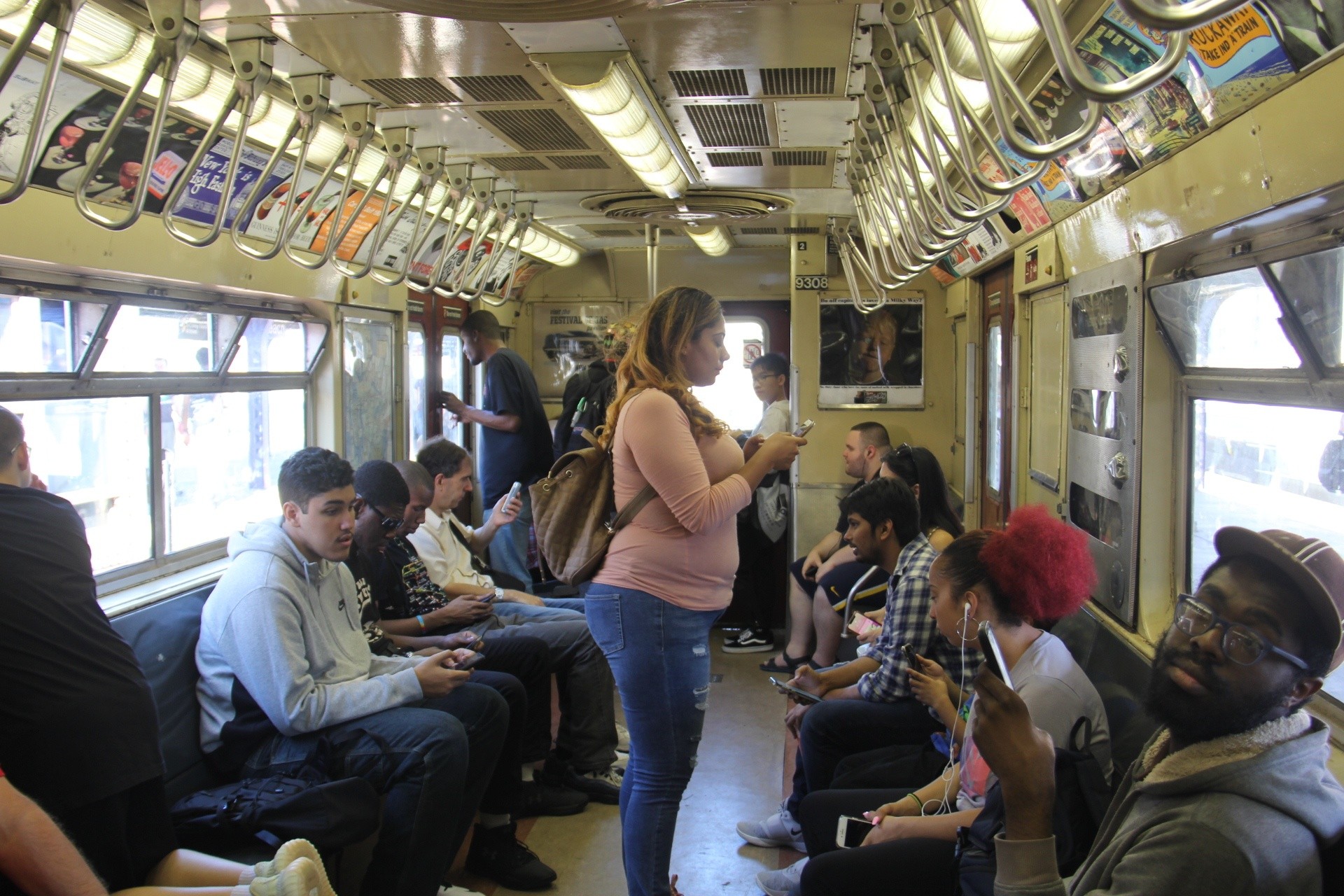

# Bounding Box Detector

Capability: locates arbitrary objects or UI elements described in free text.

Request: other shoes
[614,722,630,751]
[250,838,336,896]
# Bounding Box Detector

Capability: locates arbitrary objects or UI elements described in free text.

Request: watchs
[494,587,504,603]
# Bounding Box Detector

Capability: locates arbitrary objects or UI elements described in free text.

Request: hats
[1213,526,1343,678]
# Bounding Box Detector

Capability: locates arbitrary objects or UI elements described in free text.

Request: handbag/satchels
[479,567,525,592]
[950,714,1124,894]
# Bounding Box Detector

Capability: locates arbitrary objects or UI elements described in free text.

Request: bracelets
[907,792,924,812]
[416,615,427,636]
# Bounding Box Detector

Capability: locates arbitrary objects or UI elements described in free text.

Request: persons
[0,402,334,896]
[195,283,807,896]
[833,309,898,385]
[736,422,1344,896]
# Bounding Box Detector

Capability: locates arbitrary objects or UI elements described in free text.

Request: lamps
[527,50,700,200]
[857,0,1073,250]
[0,0,589,268]
[679,225,737,257]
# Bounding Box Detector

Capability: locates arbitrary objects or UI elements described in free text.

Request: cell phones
[977,619,1016,692]
[792,418,815,439]
[466,635,483,650]
[836,815,877,849]
[804,566,819,576]
[447,653,485,671]
[847,610,883,637]
[501,481,521,513]
[478,593,496,603]
[769,676,825,704]
[902,643,925,679]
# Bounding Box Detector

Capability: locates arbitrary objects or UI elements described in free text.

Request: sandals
[805,655,841,670]
[759,650,809,674]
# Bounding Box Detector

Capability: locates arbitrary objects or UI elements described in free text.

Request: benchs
[1045,598,1167,770]
[107,580,333,865]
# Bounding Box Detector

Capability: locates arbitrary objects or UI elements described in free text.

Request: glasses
[894,442,918,483]
[364,502,405,531]
[1173,594,1310,673]
[752,374,778,383]
[12,443,32,458]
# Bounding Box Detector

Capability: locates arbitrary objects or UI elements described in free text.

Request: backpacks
[528,384,696,585]
[166,729,394,857]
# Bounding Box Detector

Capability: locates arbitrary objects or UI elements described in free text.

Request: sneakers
[547,748,623,805]
[757,855,810,896]
[480,776,589,815]
[737,796,808,854]
[464,824,557,891]
[721,628,774,654]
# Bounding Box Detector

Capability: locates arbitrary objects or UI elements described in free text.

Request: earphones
[963,603,971,620]
